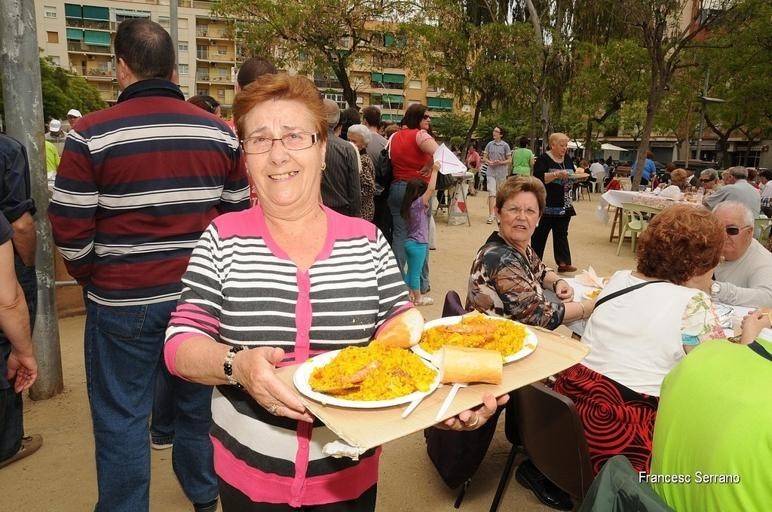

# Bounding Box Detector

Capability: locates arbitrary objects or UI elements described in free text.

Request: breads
[437,344,504,386]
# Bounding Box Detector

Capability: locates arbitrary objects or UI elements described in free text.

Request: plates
[581,289,593,300]
[413,312,536,364]
[293,347,437,410]
[572,174,590,181]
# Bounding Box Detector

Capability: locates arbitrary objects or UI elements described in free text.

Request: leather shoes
[513,456,575,511]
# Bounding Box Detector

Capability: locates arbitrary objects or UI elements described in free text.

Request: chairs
[423,290,524,512]
[605,177,622,212]
[754,218,772,246]
[616,201,664,255]
[651,174,660,192]
[588,171,608,194]
[577,182,591,202]
[576,454,675,512]
[489,383,595,512]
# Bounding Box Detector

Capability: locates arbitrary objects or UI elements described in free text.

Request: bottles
[637,180,707,207]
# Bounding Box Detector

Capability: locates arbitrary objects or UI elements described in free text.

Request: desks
[433,172,474,226]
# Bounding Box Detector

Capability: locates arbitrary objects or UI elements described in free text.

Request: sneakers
[557,265,577,273]
[151,439,174,451]
[2,431,45,471]
[486,215,494,225]
[408,285,435,307]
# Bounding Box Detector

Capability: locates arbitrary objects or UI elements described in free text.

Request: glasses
[699,176,716,183]
[240,130,319,155]
[423,114,432,120]
[725,223,751,236]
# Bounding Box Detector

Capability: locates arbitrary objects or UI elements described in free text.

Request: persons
[0,19,771,512]
